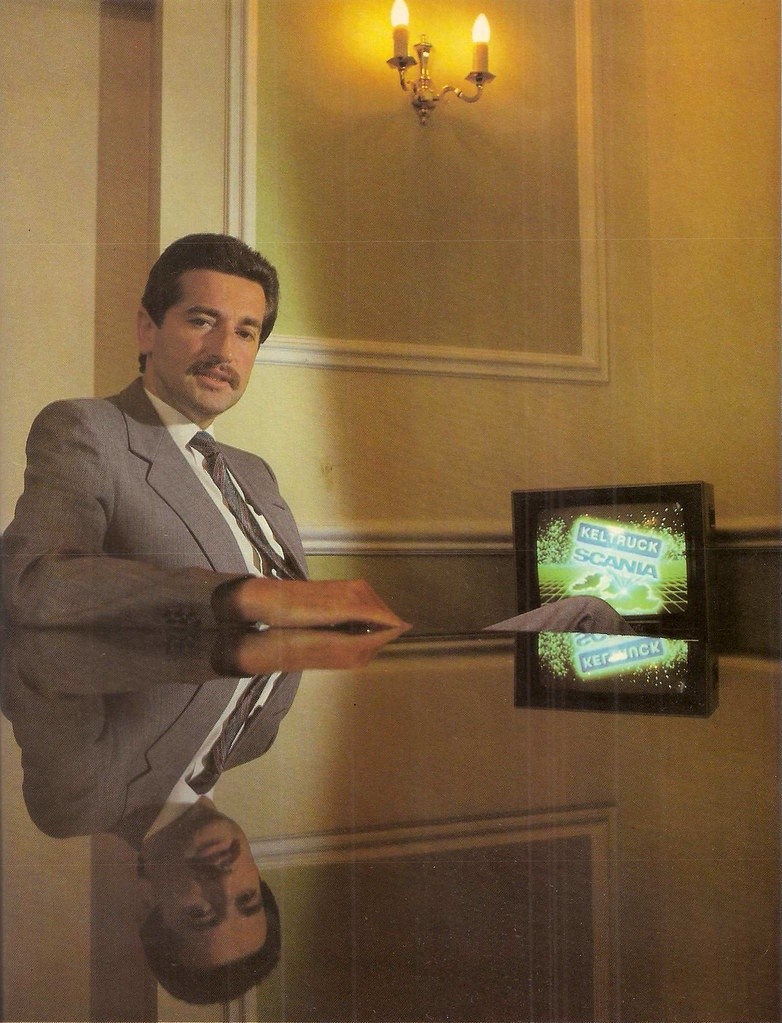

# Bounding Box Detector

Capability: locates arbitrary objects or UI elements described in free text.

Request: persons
[0,234,634,636]
[0,631,397,1007]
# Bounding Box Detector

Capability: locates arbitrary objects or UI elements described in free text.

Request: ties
[189,430,300,582]
[188,672,273,795]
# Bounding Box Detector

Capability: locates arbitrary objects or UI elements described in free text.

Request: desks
[0,629,782,1023]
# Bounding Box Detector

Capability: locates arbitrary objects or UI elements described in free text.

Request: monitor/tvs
[512,633,719,716]
[512,480,721,635]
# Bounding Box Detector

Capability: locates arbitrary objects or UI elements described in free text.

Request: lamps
[390,0,497,126]
